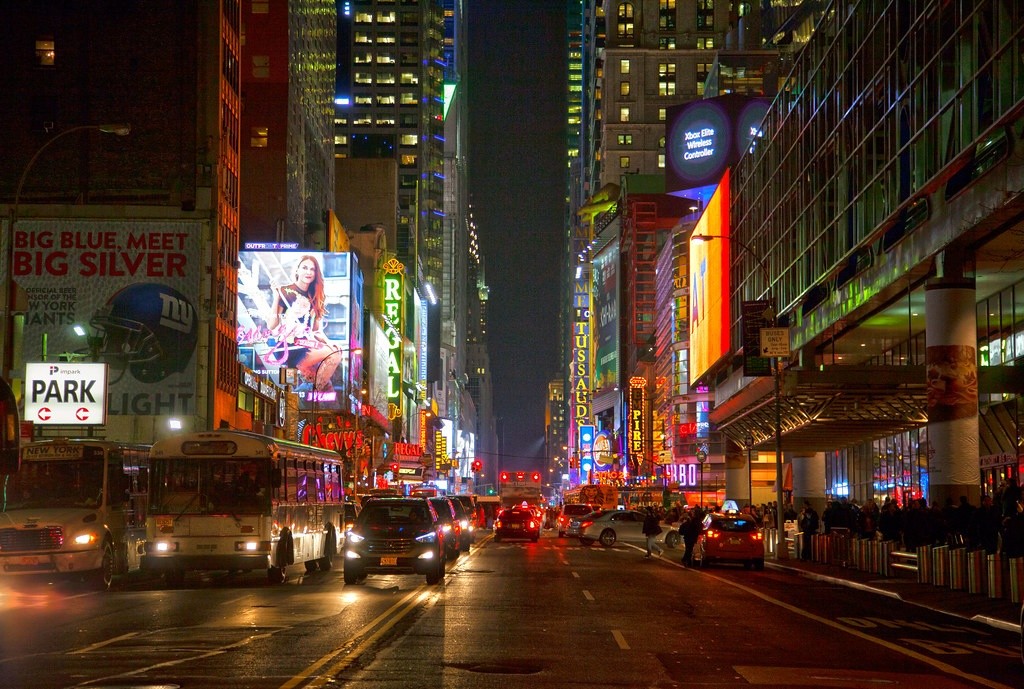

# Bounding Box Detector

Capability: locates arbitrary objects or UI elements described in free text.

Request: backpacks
[678,519,695,536]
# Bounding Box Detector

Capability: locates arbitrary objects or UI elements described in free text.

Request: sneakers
[658,548,665,557]
[644,554,652,559]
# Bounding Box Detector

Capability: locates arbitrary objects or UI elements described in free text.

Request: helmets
[88,283,199,385]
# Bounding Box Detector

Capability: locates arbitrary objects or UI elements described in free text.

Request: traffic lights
[471,461,481,473]
[569,457,574,469]
[575,457,581,469]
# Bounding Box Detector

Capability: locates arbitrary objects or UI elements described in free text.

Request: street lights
[744,435,754,513]
[309,348,362,446]
[691,234,788,556]
[696,449,707,510]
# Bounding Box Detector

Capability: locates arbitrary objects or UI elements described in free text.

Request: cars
[493,504,561,543]
[423,495,463,561]
[565,510,600,545]
[447,495,478,531]
[690,500,766,571]
[556,503,596,537]
[448,497,475,553]
[577,510,681,547]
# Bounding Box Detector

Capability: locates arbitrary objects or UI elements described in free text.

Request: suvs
[341,494,448,585]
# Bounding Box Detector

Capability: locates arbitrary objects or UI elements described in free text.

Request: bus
[1,436,153,591]
[136,426,344,587]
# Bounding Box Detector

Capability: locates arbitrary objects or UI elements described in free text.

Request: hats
[644,506,654,512]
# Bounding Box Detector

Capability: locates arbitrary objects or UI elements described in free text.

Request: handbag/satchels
[654,525,663,536]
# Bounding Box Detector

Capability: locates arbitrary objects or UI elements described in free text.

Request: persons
[266,256,344,408]
[477,488,1024,569]
